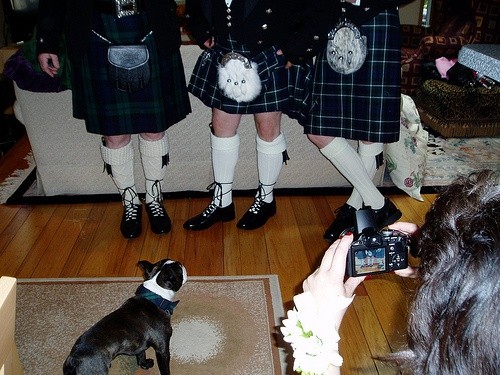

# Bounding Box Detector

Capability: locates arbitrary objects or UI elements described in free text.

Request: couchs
[400,0,489,100]
[13,40,386,197]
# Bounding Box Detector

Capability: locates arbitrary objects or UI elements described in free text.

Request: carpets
[0,123,500,204]
[14,275,295,375]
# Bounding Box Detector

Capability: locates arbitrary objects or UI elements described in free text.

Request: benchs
[416,79,500,139]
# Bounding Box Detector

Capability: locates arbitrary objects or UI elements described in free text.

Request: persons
[34,0,215,240]
[280,167,500,375]
[180,0,418,241]
[356,248,385,268]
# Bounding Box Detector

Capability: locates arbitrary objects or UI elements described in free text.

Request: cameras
[346,227,408,276]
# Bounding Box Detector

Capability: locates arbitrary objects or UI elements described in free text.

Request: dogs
[63,258,188,375]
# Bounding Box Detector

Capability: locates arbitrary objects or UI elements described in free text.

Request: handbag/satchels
[326,20,367,74]
[108,45,150,93]
[217,52,262,103]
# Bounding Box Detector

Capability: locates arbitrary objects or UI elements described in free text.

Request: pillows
[385,94,428,201]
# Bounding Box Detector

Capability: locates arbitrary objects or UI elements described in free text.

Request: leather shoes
[322,203,363,242]
[353,197,402,241]
[120,198,143,239]
[144,200,171,234]
[183,200,235,230]
[236,197,275,230]
